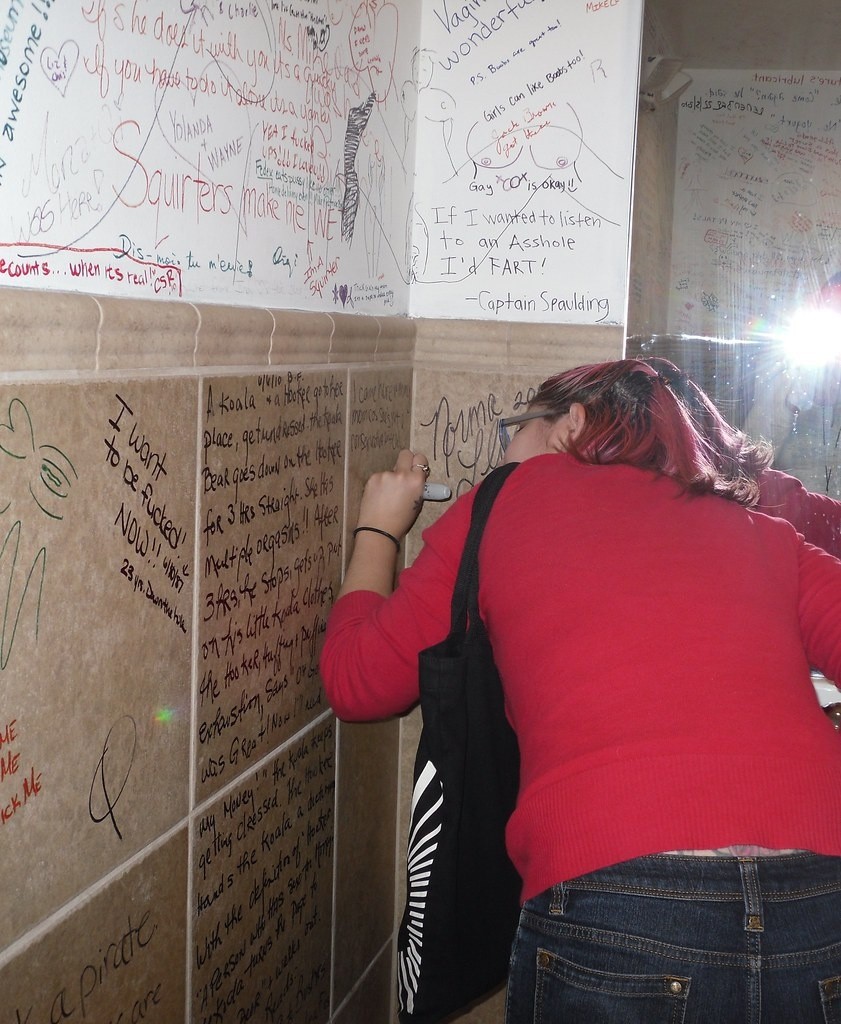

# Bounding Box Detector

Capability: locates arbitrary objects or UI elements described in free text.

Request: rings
[412,463,430,475]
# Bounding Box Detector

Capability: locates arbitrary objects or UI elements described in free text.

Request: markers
[423,483,451,502]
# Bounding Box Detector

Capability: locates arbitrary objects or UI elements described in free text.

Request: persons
[648,272,840,564]
[321,361,840,1024]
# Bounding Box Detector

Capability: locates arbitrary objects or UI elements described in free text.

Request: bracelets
[352,526,401,551]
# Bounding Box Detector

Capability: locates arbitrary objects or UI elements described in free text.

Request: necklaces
[818,384,841,496]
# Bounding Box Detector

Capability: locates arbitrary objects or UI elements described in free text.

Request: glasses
[494,407,572,451]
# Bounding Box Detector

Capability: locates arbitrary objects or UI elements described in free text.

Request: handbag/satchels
[389,461,518,1023]
